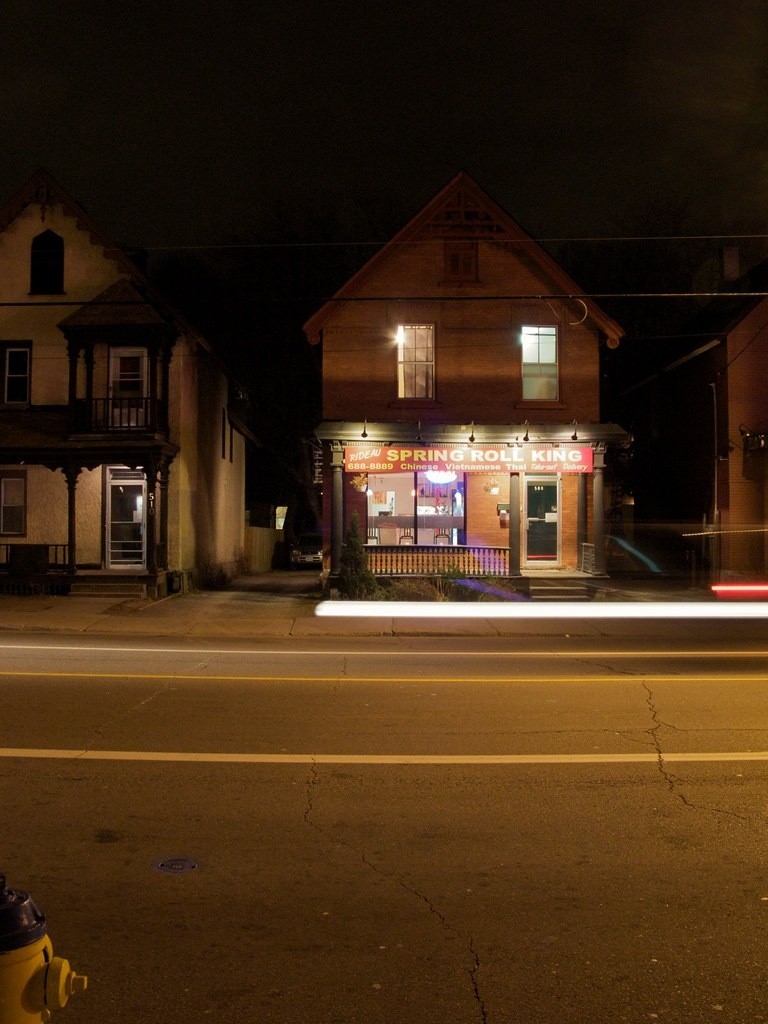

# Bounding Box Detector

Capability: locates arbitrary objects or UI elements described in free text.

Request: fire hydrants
[0,886,91,1024]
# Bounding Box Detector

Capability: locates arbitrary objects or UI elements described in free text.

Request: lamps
[362,418,577,443]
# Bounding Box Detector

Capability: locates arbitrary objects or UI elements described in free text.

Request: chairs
[367,524,450,546]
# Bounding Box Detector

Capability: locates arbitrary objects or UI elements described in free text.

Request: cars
[288,532,324,571]
